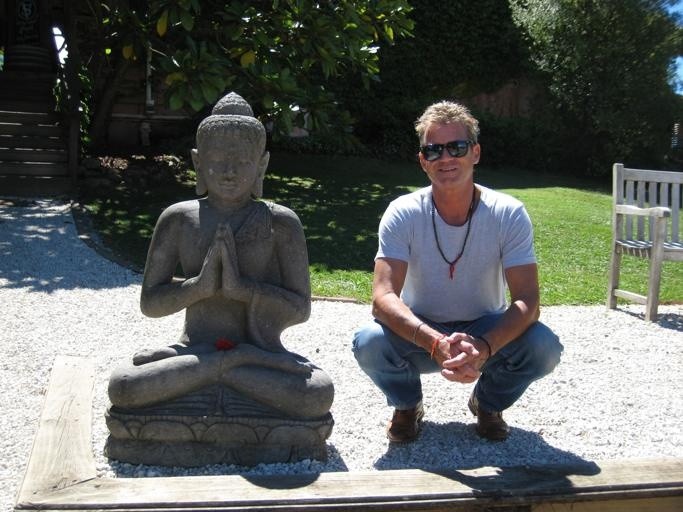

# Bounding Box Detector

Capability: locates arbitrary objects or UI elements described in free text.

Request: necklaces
[432,184,475,280]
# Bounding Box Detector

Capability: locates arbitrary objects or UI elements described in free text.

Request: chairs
[608,162,683,325]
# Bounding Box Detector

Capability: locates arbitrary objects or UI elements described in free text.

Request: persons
[107,91,335,419]
[351,100,564,443]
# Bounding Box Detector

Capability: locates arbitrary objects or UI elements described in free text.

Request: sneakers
[385,399,423,442]
[466,388,510,440]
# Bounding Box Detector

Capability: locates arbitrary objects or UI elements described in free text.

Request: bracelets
[412,321,425,345]
[477,335,492,359]
[430,333,447,359]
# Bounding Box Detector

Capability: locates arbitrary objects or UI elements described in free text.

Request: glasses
[418,135,474,162]
